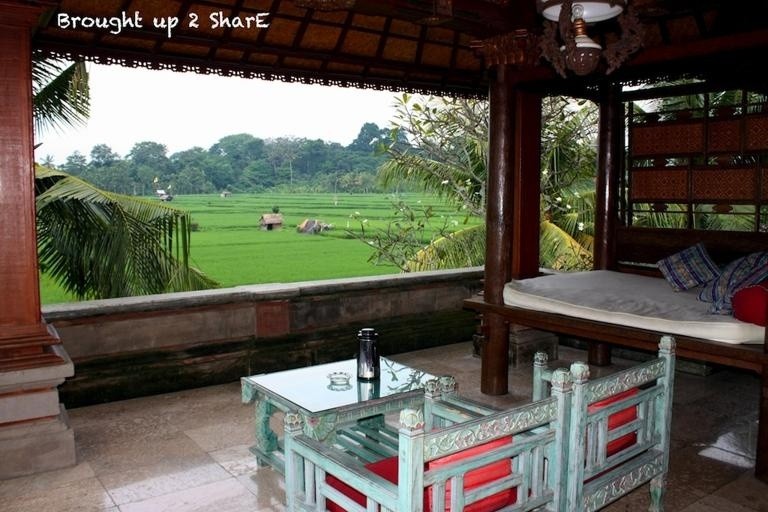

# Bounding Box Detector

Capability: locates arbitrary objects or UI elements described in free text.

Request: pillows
[428,427,512,512]
[586,386,639,458]
[657,242,768,326]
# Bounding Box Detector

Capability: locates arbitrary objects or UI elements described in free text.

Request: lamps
[537,0,624,76]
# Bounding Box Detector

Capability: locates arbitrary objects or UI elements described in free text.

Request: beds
[462,265,768,484]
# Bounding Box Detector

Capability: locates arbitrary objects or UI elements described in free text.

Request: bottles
[357,326,382,382]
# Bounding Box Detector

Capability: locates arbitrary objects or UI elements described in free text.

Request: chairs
[282,367,573,512]
[438,335,677,512]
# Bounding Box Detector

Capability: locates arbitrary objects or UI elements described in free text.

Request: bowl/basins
[328,372,353,389]
